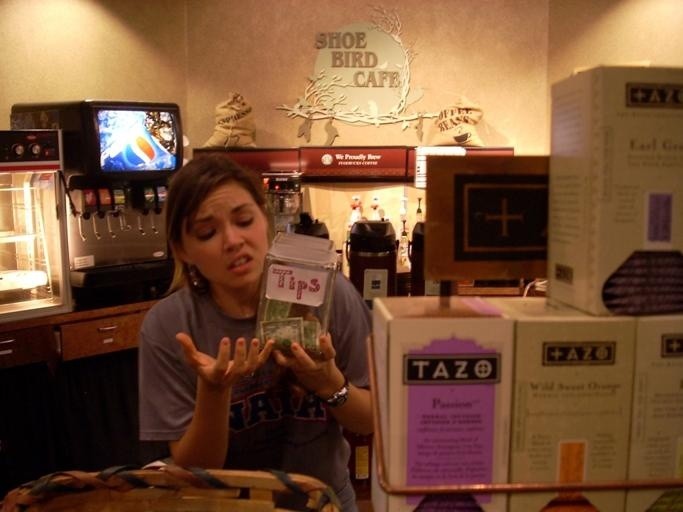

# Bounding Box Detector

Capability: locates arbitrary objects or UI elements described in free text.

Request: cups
[452,132,471,143]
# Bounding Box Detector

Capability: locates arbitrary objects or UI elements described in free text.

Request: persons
[129,148,379,512]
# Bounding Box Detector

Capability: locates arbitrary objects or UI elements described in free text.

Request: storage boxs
[546,63,682,316]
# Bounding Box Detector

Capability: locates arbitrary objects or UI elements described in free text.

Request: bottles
[347,192,423,270]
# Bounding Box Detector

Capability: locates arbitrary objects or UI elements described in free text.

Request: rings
[242,372,252,380]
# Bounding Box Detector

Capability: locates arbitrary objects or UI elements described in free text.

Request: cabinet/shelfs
[0,295,162,368]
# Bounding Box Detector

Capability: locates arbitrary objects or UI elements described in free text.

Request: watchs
[319,375,356,407]
